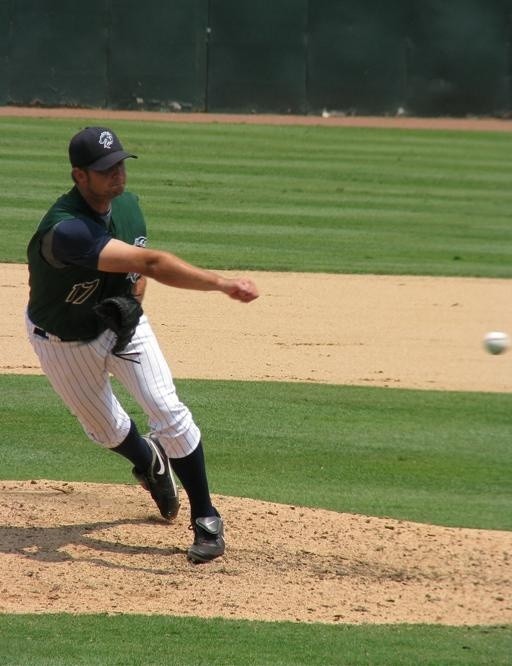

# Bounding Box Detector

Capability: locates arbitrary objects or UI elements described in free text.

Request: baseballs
[485,331,506,353]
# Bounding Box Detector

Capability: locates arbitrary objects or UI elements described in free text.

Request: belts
[34,327,47,340]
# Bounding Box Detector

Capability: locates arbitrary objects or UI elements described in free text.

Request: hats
[69,126,138,172]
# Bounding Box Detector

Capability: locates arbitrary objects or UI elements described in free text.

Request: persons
[24,127,258,562]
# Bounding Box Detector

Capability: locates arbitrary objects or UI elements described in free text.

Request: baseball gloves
[92,295,142,352]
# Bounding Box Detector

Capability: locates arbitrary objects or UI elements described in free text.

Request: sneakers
[187,505,225,562]
[132,431,181,520]
